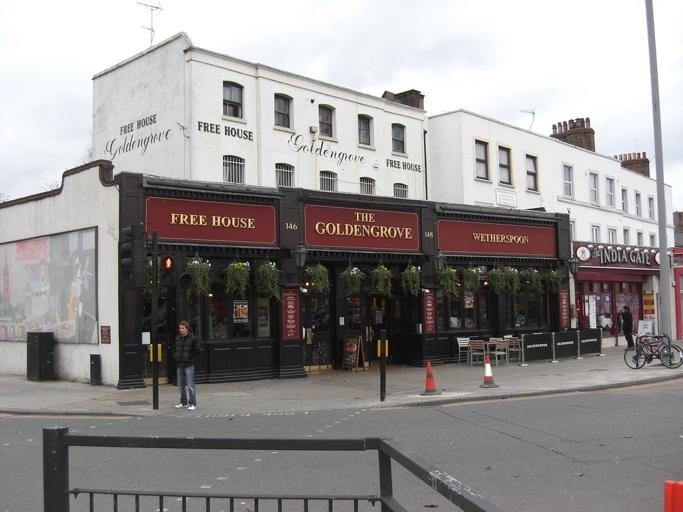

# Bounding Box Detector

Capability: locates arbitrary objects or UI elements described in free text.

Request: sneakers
[187,404,197,411]
[624,347,635,351]
[174,403,189,408]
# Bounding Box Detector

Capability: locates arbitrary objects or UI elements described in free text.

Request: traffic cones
[476,354,502,390]
[418,359,445,397]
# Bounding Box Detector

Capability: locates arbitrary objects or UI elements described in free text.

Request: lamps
[567,253,580,281]
[431,246,449,274]
[288,239,311,282]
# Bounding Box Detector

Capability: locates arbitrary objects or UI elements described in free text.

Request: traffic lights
[175,272,193,289]
[157,253,177,290]
[119,222,146,292]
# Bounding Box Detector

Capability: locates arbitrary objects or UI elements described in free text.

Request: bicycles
[623,331,683,371]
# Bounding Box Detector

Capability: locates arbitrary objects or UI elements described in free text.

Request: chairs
[456,334,522,367]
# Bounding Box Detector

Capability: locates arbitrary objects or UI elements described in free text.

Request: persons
[170,320,200,410]
[619,305,635,351]
